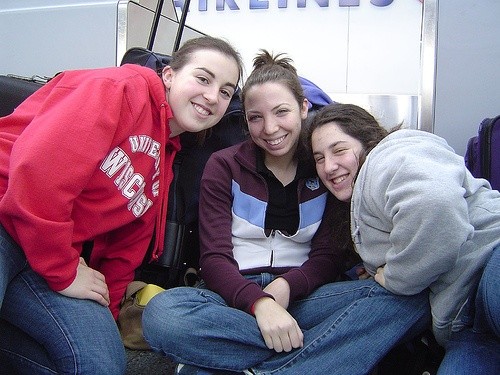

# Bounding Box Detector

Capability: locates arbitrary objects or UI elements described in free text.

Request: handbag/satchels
[118,165,185,351]
[121,48,244,111]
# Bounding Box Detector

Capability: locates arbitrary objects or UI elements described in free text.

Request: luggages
[464,115,500,191]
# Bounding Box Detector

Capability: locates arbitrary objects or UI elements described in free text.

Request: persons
[0,36,246,375]
[306,103,500,374]
[142,50,430,375]
